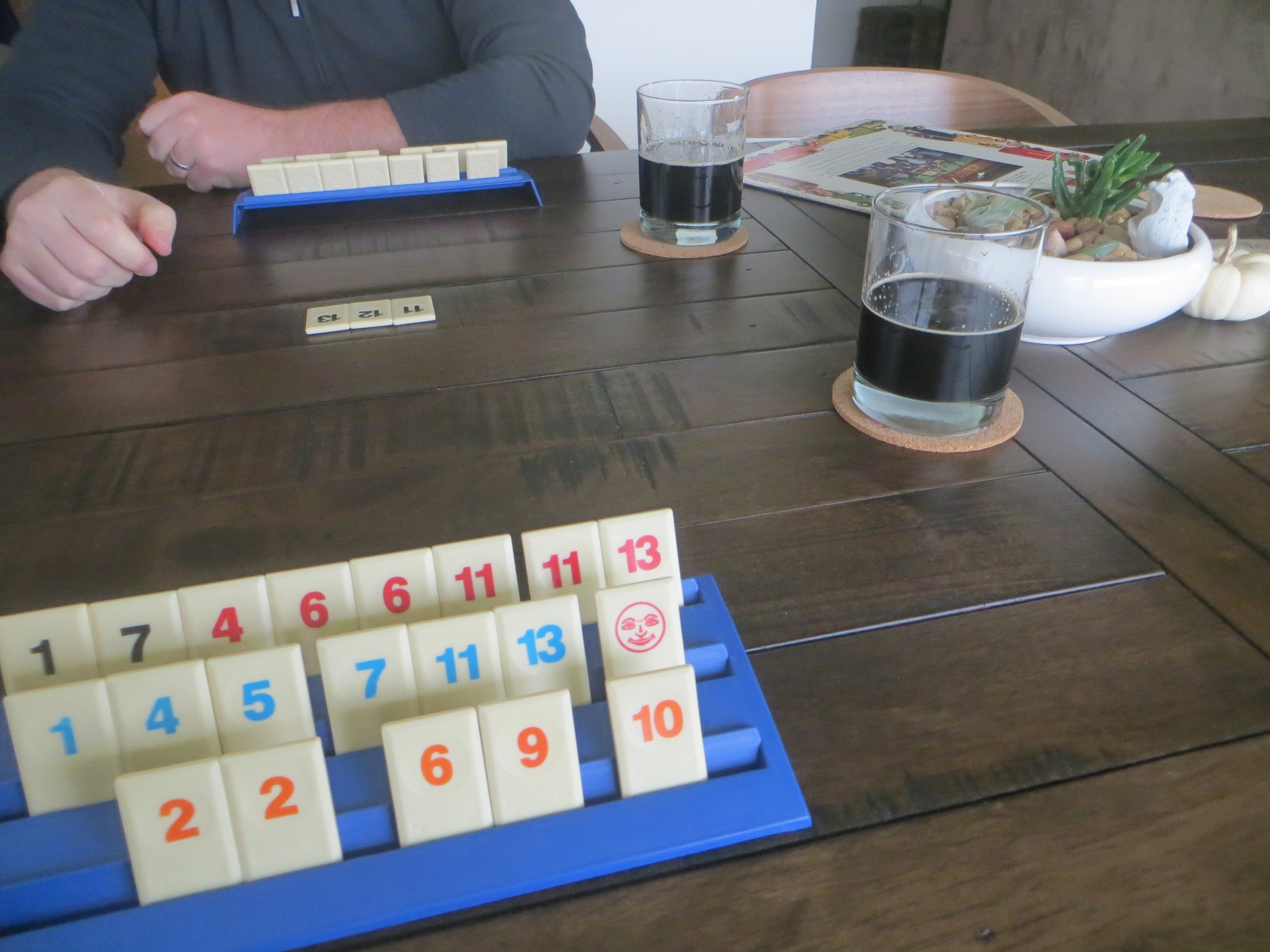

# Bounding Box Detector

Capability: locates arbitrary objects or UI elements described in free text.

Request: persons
[0,0,598,321]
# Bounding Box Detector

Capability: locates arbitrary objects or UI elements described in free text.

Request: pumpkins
[1184,224,1270,320]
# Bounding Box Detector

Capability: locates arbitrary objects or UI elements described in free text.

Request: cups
[854,186,1050,435]
[632,80,751,243]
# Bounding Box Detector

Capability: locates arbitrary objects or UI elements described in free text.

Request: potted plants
[900,134,1216,346]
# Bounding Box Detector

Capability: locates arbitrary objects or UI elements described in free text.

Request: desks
[1,111,1270,952]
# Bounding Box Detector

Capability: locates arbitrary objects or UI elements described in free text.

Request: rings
[167,152,189,172]
